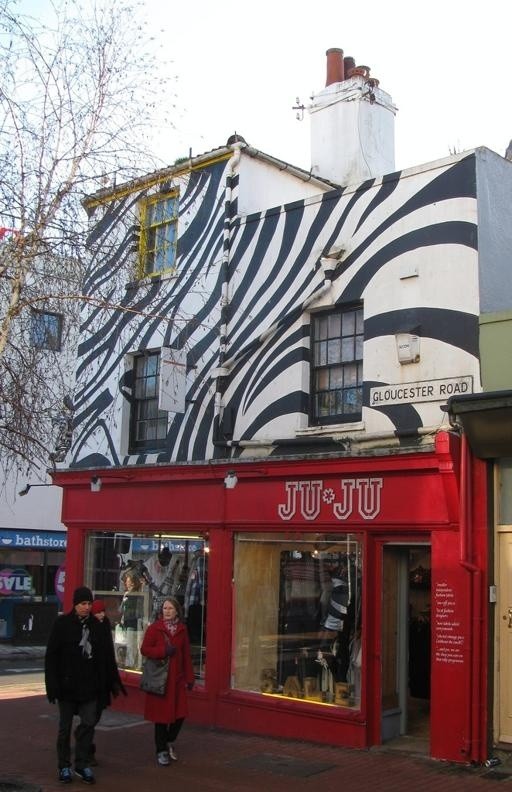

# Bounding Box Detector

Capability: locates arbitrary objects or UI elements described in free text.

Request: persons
[119,575,141,669]
[139,595,195,765]
[45,585,115,785]
[91,599,127,765]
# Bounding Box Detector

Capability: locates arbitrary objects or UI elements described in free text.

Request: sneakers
[73,757,97,784]
[157,742,178,765]
[57,767,73,784]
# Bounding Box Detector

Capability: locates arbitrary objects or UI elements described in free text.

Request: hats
[74,587,93,602]
[91,600,105,615]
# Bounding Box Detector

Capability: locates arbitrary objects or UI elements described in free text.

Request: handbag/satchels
[140,655,170,695]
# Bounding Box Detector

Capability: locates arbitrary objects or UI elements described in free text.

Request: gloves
[166,644,176,655]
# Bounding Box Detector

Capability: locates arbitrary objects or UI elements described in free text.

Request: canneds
[484,757,499,768]
